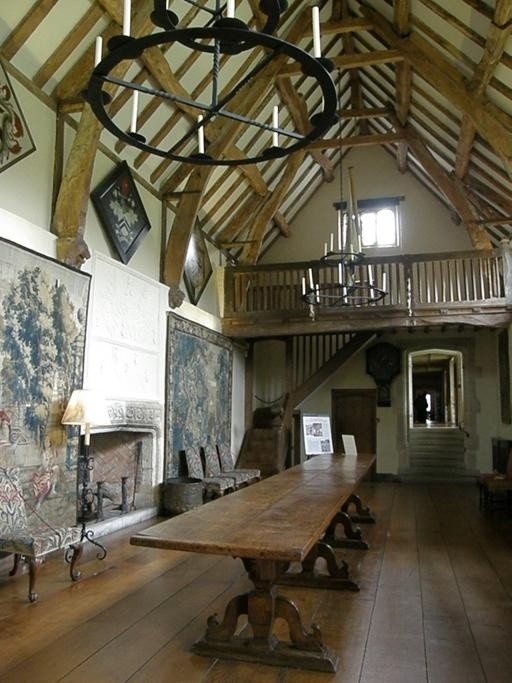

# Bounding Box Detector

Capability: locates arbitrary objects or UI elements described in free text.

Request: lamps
[60,388,113,563]
[82,1,340,168]
[297,165,392,311]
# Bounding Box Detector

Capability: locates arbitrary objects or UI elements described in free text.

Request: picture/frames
[90,160,153,266]
[1,64,37,177]
[182,215,215,304]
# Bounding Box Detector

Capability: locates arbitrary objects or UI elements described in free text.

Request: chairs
[183,438,263,498]
[477,440,511,520]
[0,468,84,605]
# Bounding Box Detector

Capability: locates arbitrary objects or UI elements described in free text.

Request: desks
[128,451,380,675]
